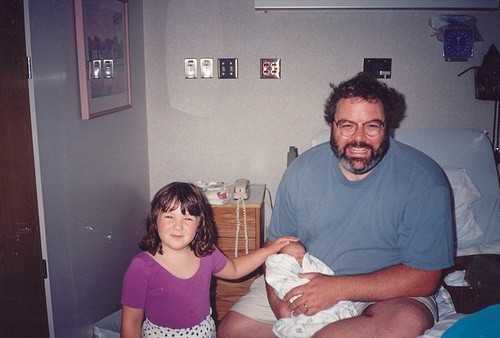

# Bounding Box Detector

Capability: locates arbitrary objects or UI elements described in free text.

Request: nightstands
[203,182,267,321]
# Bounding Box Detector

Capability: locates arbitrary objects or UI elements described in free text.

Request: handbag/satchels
[440,253,500,313]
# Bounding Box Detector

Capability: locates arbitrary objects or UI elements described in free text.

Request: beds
[287,126,500,338]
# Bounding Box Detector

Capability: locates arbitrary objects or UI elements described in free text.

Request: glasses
[334,115,386,137]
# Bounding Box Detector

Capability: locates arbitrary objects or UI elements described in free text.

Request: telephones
[233,178,250,200]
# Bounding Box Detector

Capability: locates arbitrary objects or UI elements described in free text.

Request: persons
[217,72,456,338]
[264,241,358,338]
[120,182,300,338]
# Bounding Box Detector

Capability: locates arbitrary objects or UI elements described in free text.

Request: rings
[304,304,309,309]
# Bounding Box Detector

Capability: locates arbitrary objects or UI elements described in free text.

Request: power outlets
[259,58,281,80]
[184,58,198,79]
[362,58,392,79]
[200,58,213,79]
[216,58,239,80]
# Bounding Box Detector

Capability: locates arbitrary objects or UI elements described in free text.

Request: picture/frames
[73,0,133,120]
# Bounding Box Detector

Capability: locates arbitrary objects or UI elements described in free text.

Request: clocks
[442,25,475,58]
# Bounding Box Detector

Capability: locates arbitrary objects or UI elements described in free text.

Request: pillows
[438,165,483,241]
